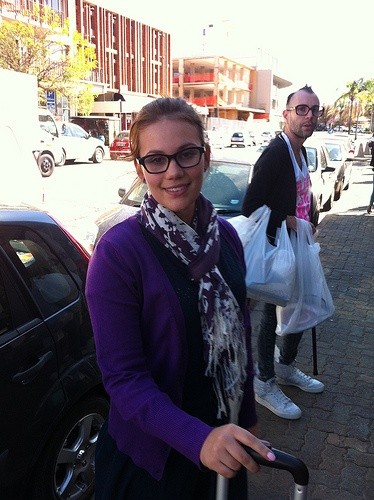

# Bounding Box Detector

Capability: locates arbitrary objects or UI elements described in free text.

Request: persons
[367,133,374,214]
[242,84,325,420]
[85,97,276,500]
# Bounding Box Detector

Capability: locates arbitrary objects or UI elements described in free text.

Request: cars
[316,126,331,131]
[303,135,354,226]
[56,121,110,166]
[231,131,281,148]
[333,125,349,131]
[0,210,110,500]
[93,160,253,252]
[350,126,365,133]
[110,130,134,160]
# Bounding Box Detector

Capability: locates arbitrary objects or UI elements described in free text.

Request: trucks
[0,69,63,177]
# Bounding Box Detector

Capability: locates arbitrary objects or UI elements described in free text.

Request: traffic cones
[355,142,365,158]
[363,142,371,155]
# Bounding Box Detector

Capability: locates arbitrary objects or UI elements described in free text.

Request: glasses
[136,147,204,174]
[286,104,324,117]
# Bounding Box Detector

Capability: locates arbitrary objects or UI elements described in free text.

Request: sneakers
[255,384,302,420]
[278,367,325,393]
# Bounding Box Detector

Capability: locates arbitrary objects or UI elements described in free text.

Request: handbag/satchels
[275,221,335,336]
[227,204,296,305]
